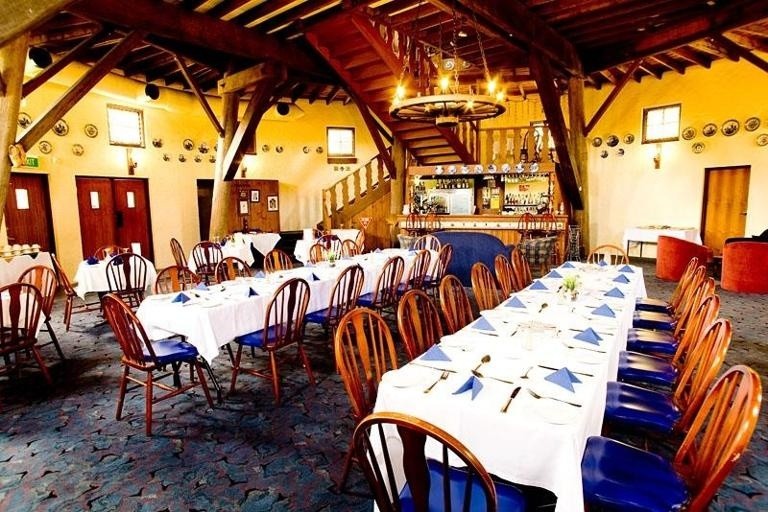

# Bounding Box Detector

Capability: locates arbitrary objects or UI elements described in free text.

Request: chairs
[581,257,762,512]
[49,252,76,332]
[335,245,649,512]
[93,245,130,260]
[403,212,421,237]
[1,282,50,384]
[424,213,442,235]
[103,294,212,436]
[105,252,146,307]
[516,212,537,246]
[519,129,539,163]
[18,265,64,359]
[129,229,454,405]
[539,213,561,267]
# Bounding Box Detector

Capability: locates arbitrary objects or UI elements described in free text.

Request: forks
[528,388,582,409]
[425,372,450,395]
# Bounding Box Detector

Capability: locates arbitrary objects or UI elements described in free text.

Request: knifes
[538,364,596,380]
[502,387,525,415]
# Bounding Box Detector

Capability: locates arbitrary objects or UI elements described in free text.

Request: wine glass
[102,247,129,263]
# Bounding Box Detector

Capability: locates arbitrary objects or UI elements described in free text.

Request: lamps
[390,1,506,128]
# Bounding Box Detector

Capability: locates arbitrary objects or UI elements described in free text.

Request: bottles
[560,203,565,214]
[505,194,541,213]
[436,177,469,189]
[422,197,446,212]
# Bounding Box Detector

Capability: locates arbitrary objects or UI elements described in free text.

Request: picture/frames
[266,195,279,212]
[250,189,260,203]
[238,199,251,216]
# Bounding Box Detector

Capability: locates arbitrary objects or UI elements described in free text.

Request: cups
[3,242,41,257]
[184,282,198,302]
[213,236,234,248]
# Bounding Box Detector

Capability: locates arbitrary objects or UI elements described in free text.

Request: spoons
[472,352,514,384]
[507,264,626,355]
[519,364,532,382]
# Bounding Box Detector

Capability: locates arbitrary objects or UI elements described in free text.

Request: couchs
[656,236,712,282]
[429,232,514,286]
[722,242,768,293]
